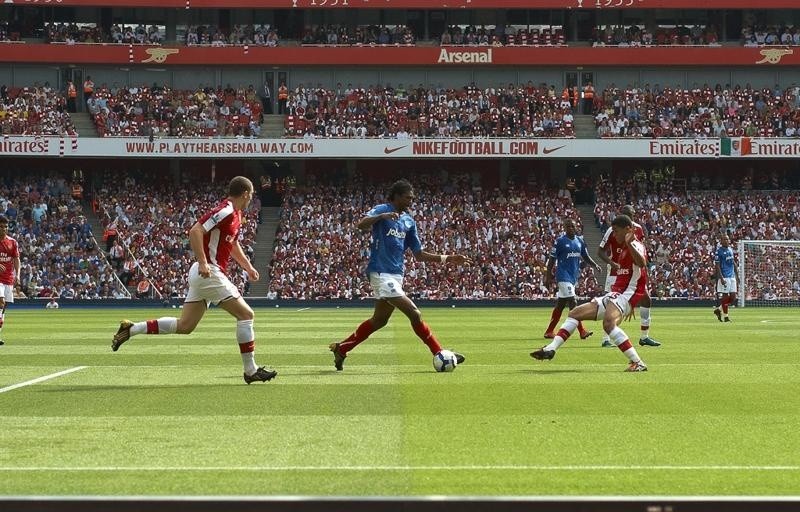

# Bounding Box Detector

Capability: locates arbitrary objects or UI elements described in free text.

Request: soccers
[433,349,458,372]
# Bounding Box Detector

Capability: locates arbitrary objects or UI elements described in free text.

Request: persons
[526,204,664,374]
[2,17,795,44]
[2,156,796,303]
[328,177,475,371]
[110,174,279,386]
[0,216,23,346]
[2,60,796,138]
[712,235,740,323]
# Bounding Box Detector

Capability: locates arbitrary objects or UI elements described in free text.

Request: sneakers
[111,320,134,352]
[544,332,557,338]
[244,366,277,384]
[454,353,465,364]
[624,360,647,371]
[329,343,347,370]
[0,341,4,345]
[714,309,723,322]
[602,340,617,347]
[580,331,593,339]
[639,336,661,346]
[724,317,730,322]
[530,345,555,361]
